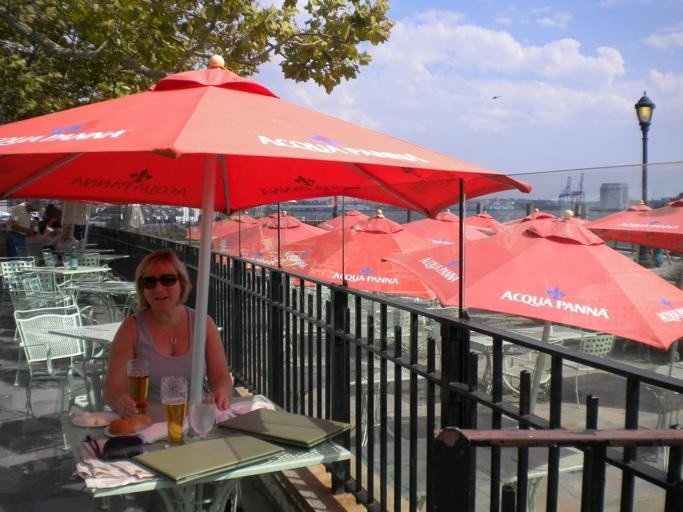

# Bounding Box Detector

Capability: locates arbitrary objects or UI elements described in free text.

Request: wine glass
[190,392,216,442]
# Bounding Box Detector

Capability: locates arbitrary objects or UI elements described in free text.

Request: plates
[71,412,147,437]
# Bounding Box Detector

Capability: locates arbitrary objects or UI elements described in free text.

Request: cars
[0,199,200,238]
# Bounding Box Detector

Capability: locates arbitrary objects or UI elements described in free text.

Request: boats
[268,199,372,213]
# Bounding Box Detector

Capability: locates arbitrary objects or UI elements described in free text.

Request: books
[131,431,285,488]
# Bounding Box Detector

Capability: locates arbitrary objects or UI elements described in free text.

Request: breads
[110,415,152,434]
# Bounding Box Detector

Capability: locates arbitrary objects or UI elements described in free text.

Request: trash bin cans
[0,231,27,261]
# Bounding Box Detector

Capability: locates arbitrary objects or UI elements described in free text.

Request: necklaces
[167,334,178,346]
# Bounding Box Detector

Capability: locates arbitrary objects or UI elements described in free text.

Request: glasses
[140,274,178,290]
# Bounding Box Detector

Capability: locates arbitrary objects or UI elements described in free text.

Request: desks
[64,392,352,512]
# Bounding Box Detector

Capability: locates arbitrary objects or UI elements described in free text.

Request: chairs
[0,245,143,419]
[357,293,615,405]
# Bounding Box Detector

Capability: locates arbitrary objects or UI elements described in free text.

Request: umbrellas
[0,49,532,220]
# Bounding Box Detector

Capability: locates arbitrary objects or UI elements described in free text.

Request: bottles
[70,244,77,270]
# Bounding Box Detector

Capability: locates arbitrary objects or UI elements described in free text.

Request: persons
[0,197,76,265]
[101,247,231,421]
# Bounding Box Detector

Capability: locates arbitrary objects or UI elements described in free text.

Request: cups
[160,377,189,441]
[49,254,57,270]
[127,359,150,416]
[61,253,70,272]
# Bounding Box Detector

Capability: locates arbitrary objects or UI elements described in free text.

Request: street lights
[634,87,656,270]
